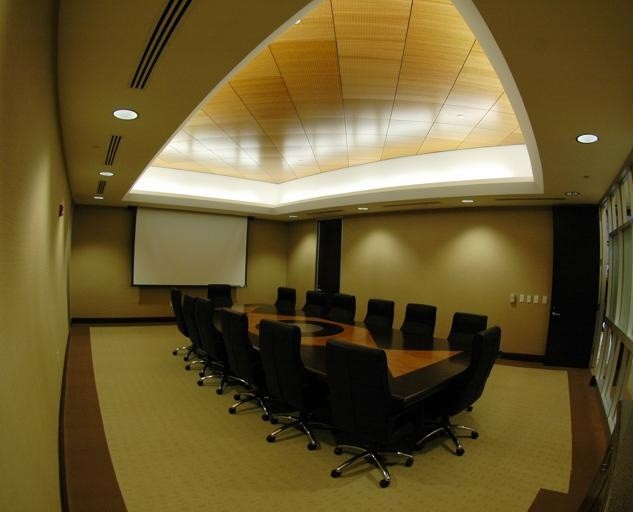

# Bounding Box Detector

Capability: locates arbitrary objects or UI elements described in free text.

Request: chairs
[186,298,223,377]
[451,312,488,336]
[208,284,233,306]
[259,319,343,451]
[326,294,357,321]
[414,326,501,457]
[274,287,296,308]
[222,308,270,421]
[302,290,328,314]
[400,303,436,338]
[365,299,395,328]
[171,288,202,362]
[196,297,241,395]
[182,294,200,350]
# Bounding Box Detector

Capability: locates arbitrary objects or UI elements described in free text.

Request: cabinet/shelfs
[588,175,633,449]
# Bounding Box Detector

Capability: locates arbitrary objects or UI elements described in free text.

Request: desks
[217,303,472,441]
